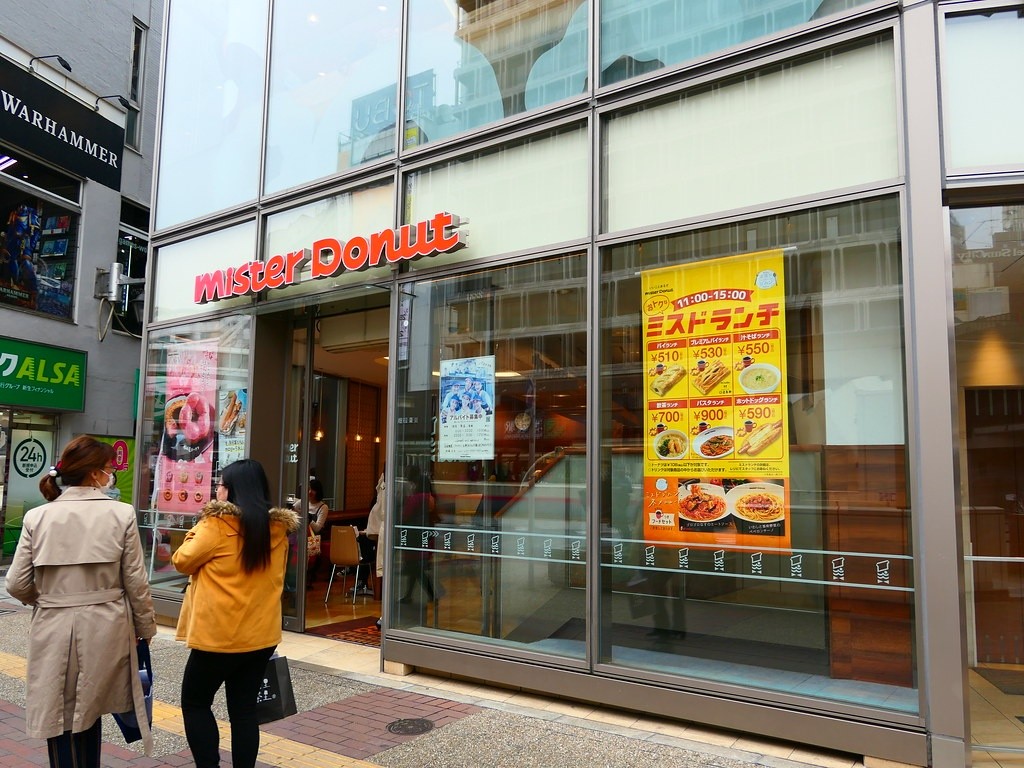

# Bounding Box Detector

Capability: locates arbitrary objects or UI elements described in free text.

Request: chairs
[323,525,374,605]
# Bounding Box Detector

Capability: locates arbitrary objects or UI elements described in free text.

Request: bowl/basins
[740,364,780,395]
[654,430,688,460]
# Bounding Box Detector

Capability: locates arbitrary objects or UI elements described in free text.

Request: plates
[725,481,785,523]
[693,427,734,459]
[677,484,730,524]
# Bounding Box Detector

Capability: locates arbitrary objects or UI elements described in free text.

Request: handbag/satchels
[254,657,297,725]
[110,639,155,742]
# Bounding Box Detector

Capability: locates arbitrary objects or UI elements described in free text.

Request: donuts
[165,393,212,444]
[163,471,204,502]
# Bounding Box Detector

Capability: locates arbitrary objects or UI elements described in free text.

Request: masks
[95,469,115,494]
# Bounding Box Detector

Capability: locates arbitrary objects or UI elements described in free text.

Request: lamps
[28,53,72,77]
[95,262,146,322]
[95,93,142,116]
[312,427,381,446]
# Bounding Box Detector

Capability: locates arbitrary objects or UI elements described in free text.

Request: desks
[326,509,371,522]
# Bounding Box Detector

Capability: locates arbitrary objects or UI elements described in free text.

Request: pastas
[735,491,786,523]
[677,485,728,521]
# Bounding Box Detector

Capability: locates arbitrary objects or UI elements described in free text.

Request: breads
[649,362,686,396]
[221,392,244,432]
[692,360,732,396]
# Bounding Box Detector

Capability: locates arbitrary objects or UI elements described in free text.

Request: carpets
[303,615,381,648]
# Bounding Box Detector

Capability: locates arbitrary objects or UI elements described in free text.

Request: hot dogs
[738,418,783,456]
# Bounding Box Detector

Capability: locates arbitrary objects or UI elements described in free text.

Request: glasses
[103,467,118,473]
[214,483,225,489]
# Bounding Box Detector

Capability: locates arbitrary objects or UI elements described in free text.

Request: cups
[655,364,666,375]
[743,356,755,367]
[744,421,757,433]
[657,423,667,432]
[697,361,708,371]
[699,423,708,434]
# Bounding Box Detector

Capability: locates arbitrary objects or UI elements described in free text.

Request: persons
[629,504,689,638]
[336,554,374,592]
[365,471,386,629]
[170,459,303,768]
[397,474,440,604]
[4,435,157,768]
[292,479,329,589]
